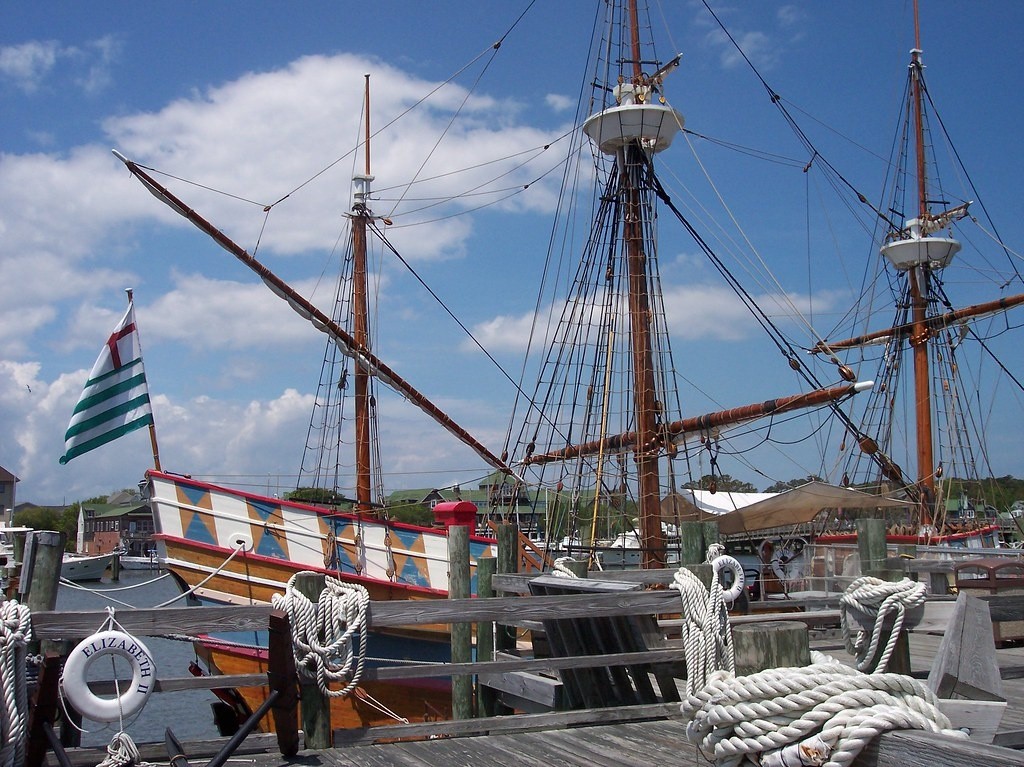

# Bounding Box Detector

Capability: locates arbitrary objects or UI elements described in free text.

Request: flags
[58,300,153,465]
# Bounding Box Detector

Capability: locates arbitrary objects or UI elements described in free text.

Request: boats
[60,551,114,582]
[118,555,160,570]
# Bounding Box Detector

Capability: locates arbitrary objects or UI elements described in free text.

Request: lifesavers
[61,631,157,723]
[771,550,799,580]
[711,555,745,602]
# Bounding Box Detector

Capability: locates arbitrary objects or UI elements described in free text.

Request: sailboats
[104,1,1024,754]
